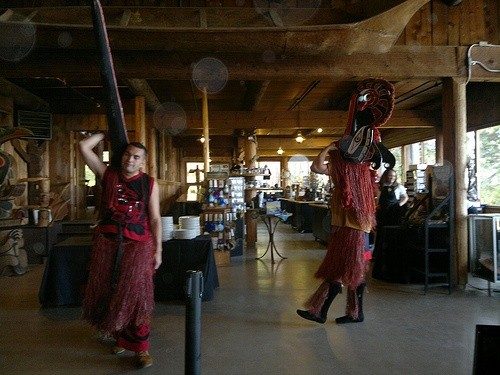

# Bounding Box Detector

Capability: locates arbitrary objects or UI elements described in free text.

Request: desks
[276,197,330,247]
[37,234,220,309]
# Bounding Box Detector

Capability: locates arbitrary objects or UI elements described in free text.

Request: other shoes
[112,344,125,354]
[135,350,152,368]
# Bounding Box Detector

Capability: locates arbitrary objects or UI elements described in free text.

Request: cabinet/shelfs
[401,165,456,297]
[204,173,273,247]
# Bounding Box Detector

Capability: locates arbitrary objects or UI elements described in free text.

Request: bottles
[204,180,238,221]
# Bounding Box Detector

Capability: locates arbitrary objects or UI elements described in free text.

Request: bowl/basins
[204,221,224,231]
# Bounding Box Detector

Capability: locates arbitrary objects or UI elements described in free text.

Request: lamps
[292,103,307,143]
[275,129,286,155]
[196,129,211,143]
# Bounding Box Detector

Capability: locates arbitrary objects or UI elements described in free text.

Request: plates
[160,217,202,242]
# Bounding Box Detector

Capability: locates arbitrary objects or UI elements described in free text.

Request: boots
[335,283,366,324]
[296,280,342,324]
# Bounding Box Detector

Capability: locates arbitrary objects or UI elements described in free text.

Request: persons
[296,142,380,324]
[368,168,409,262]
[79,131,163,368]
